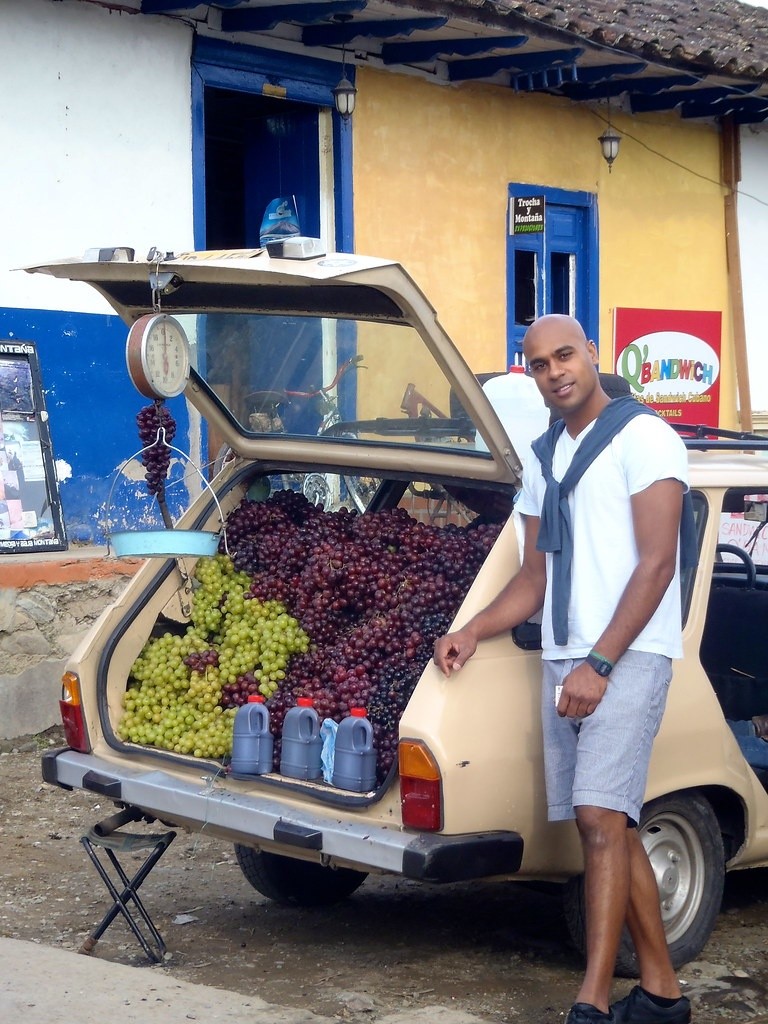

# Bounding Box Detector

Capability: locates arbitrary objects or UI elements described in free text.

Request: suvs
[9,236,768,977]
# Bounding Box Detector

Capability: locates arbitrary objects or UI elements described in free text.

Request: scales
[104,246,238,559]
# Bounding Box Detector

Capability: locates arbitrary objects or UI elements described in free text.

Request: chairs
[702,588,768,720]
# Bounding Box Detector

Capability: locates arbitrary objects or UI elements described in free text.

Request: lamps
[333,44,358,128]
[598,82,620,175]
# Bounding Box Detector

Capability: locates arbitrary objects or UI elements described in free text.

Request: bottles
[280,698,323,780]
[230,695,272,775]
[332,708,378,792]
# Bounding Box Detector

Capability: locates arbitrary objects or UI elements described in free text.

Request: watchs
[587,654,612,677]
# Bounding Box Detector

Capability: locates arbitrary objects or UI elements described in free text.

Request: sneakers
[610,985,691,1024]
[564,1003,617,1024]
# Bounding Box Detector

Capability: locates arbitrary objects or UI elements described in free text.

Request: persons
[433,313,695,1024]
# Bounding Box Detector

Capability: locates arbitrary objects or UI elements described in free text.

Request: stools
[70,825,180,962]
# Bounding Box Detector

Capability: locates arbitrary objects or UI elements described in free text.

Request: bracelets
[590,650,613,668]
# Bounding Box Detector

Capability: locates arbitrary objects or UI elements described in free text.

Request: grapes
[118,490,506,790]
[137,404,177,496]
